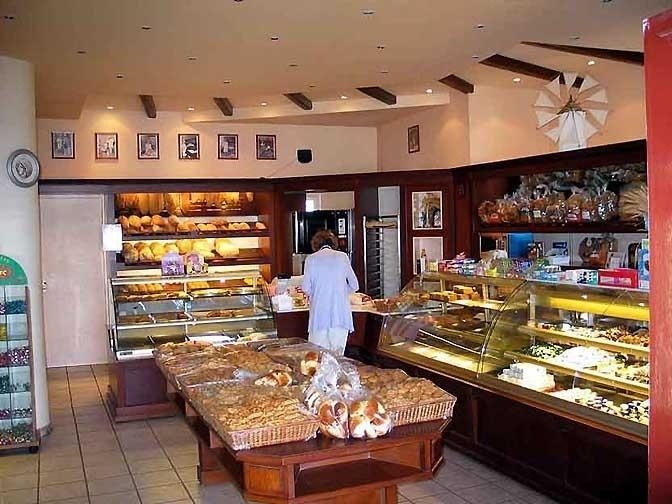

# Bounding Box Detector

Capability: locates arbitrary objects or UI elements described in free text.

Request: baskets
[376,367,457,426]
[183,380,320,450]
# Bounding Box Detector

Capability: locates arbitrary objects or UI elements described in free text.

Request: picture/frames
[93,130,120,161]
[49,128,76,162]
[135,131,159,160]
[176,132,201,161]
[216,132,239,161]
[407,124,421,154]
[254,132,278,161]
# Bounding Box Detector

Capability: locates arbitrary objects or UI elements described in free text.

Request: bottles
[420,248,427,266]
[527,242,537,258]
[536,243,543,258]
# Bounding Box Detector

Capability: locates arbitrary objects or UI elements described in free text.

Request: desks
[160,372,451,503]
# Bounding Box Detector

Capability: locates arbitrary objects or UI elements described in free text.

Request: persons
[302,228,359,356]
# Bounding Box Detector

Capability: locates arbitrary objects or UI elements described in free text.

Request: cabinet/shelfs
[374,269,649,446]
[107,190,278,423]
[0,284,44,457]
[476,221,649,270]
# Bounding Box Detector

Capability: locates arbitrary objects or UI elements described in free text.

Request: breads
[255,350,392,439]
[435,310,479,331]
[116,213,268,302]
[431,284,481,301]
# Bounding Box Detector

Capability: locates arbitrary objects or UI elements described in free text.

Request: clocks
[5,148,41,187]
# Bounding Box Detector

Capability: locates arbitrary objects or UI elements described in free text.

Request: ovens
[291,209,350,276]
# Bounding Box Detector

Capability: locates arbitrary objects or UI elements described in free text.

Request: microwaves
[478,232,535,258]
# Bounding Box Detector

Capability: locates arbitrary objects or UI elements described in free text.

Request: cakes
[499,320,650,426]
